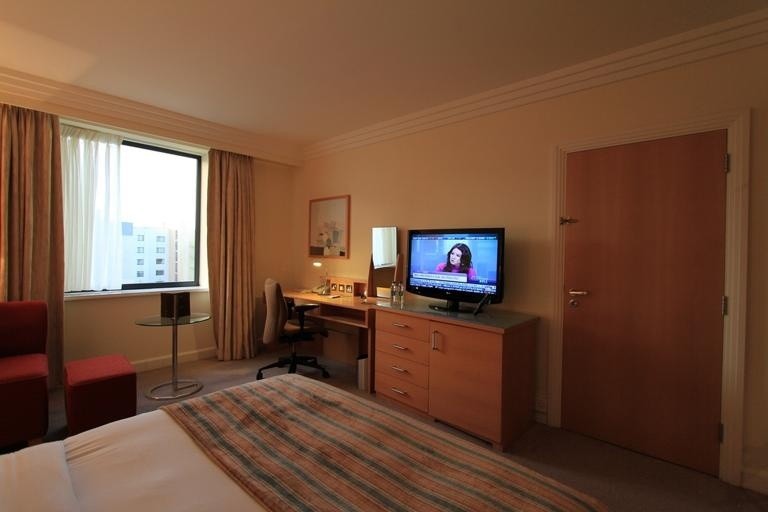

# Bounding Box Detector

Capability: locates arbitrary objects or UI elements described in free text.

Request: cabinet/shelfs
[281,289,388,393]
[375,300,540,452]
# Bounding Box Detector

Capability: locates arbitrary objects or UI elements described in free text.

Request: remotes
[472,293,491,317]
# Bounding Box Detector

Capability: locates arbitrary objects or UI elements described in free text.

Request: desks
[135,312,212,399]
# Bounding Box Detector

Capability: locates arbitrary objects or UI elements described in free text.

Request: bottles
[390,281,397,308]
[397,281,404,309]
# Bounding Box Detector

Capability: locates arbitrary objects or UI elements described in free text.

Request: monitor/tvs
[405,227,505,313]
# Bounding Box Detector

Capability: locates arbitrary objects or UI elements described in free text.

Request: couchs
[64,354,138,432]
[0,302,49,452]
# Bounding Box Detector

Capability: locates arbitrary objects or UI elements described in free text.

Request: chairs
[257,279,331,381]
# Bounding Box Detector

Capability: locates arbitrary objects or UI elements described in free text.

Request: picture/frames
[307,195,352,262]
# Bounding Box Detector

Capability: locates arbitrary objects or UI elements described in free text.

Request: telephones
[301,283,330,294]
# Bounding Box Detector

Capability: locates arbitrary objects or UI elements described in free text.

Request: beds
[0,375,609,512]
